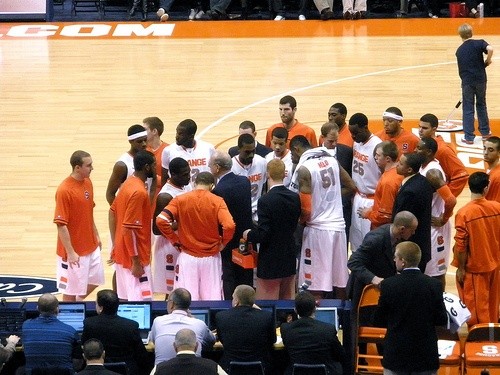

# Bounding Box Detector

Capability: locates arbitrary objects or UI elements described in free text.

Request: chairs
[353,282,500,375]
[294,363,327,375]
[229,361,266,375]
[104,362,130,375]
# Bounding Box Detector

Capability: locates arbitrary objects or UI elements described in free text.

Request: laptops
[117,302,153,346]
[56,301,85,342]
[190,306,211,329]
[0,307,28,346]
[312,306,339,334]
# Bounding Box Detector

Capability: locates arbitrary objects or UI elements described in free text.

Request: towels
[290,147,336,193]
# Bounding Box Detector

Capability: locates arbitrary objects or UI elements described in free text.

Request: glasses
[393,256,397,259]
[166,300,172,303]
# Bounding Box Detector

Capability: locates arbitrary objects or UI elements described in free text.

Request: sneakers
[189,8,219,20]
[157,8,169,21]
[142,14,148,21]
[461,139,473,144]
[274,15,286,20]
[298,15,306,20]
[129,8,135,16]
[482,131,492,140]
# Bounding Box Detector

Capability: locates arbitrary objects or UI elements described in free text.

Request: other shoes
[321,9,335,19]
[429,13,438,18]
[469,11,480,18]
[343,11,361,20]
[397,13,408,18]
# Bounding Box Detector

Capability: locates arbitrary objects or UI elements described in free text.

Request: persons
[216,284,277,375]
[151,288,217,366]
[0,335,20,372]
[346,211,418,371]
[450,171,500,328]
[483,135,500,204]
[21,293,85,375]
[77,338,118,375]
[374,242,454,375]
[82,289,150,375]
[150,328,228,375]
[455,23,493,144]
[129,0,368,21]
[280,291,344,375]
[106,95,469,301]
[52,151,104,301]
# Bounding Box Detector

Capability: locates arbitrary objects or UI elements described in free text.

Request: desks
[0,300,346,333]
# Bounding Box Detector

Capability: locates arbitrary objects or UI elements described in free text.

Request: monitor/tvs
[259,304,275,325]
